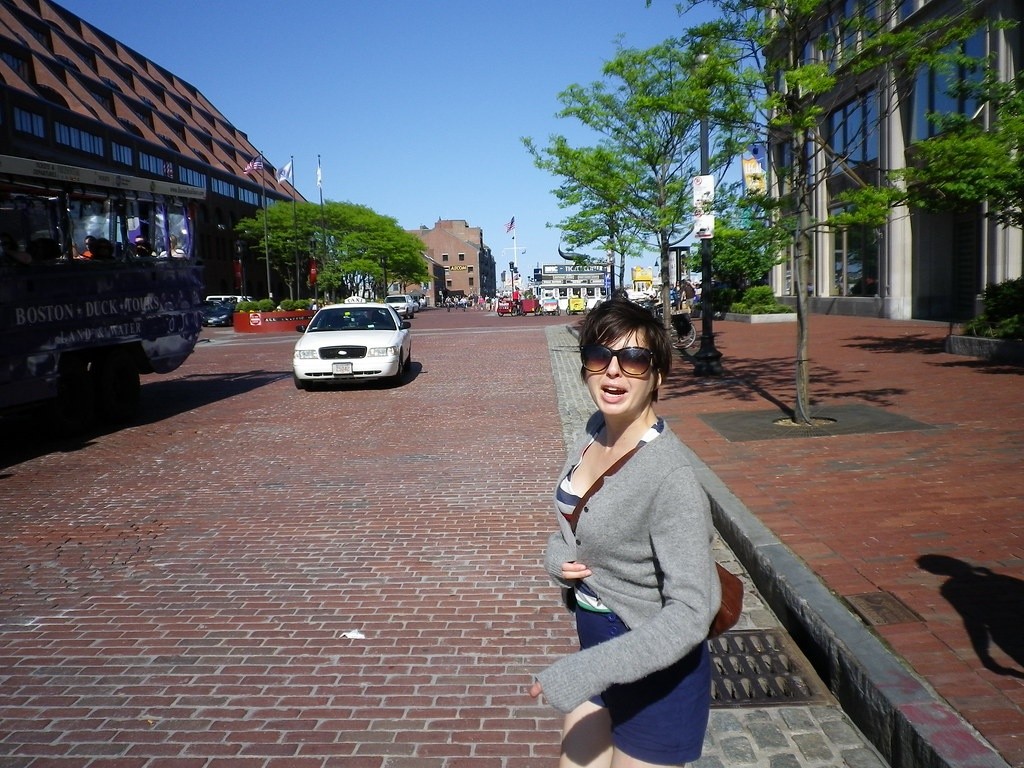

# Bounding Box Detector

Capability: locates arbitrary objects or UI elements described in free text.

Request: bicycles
[634,291,697,350]
[444,300,469,312]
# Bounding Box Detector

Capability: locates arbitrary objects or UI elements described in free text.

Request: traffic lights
[510,262,514,269]
[514,267,518,273]
[501,273,505,281]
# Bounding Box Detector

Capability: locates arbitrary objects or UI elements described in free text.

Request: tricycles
[542,298,561,315]
[566,295,587,315]
[497,296,519,317]
[519,296,542,317]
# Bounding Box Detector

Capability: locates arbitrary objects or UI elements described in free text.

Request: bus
[0,156,204,424]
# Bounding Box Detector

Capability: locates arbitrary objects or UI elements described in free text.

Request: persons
[531,303,722,768]
[584,294,589,307]
[445,295,496,311]
[77,236,156,259]
[160,236,185,257]
[513,288,523,310]
[646,280,701,313]
[0,233,56,263]
[535,305,543,316]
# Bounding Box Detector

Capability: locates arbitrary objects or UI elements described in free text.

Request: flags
[317,167,322,186]
[505,216,515,233]
[244,155,264,174]
[276,161,293,182]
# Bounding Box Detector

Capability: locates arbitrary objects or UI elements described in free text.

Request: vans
[206,295,255,305]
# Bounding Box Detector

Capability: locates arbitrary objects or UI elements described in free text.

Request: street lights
[691,43,726,378]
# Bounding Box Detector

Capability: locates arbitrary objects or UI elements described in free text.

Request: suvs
[383,294,414,320]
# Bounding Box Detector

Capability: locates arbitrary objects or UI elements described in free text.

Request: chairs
[327,316,346,330]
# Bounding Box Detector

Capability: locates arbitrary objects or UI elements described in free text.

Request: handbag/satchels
[707,561,744,639]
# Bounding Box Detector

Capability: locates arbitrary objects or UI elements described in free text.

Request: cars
[292,297,412,390]
[201,302,236,327]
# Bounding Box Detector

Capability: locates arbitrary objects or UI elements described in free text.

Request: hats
[580,344,655,375]
